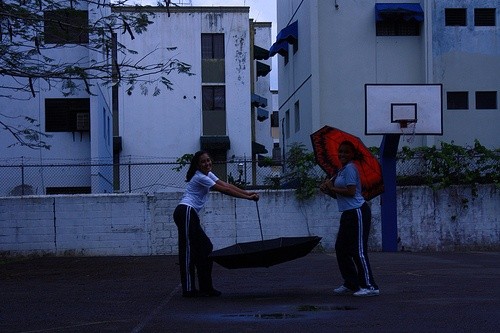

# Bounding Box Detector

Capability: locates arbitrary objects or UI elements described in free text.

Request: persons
[208,198,321,269]
[320,140,380,297]
[173,151,259,295]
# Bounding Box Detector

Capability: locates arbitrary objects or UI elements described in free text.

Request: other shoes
[199,288,223,297]
[182,290,208,298]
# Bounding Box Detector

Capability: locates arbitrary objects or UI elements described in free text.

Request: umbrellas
[310,125,385,202]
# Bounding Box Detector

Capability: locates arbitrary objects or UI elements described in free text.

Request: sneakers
[354,285,383,297]
[333,285,362,297]
